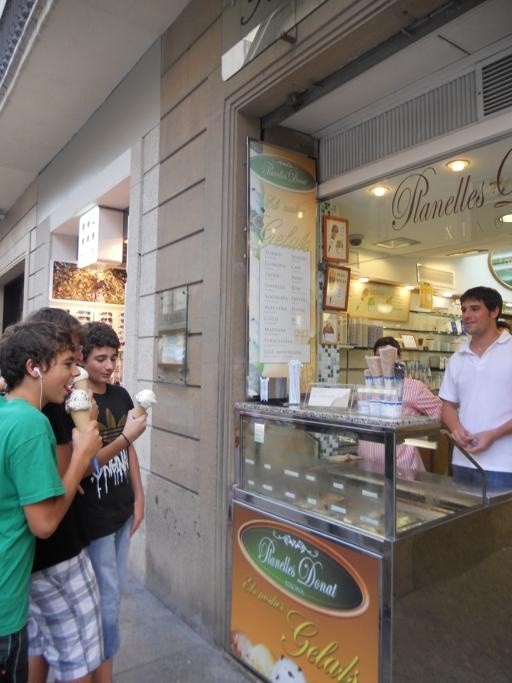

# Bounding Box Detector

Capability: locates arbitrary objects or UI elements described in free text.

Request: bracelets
[120,429,134,449]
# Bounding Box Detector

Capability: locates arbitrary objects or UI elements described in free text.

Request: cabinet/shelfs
[231,400,511,683]
[336,310,468,394]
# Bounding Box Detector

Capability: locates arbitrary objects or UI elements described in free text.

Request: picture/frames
[323,263,351,312]
[348,280,411,323]
[320,310,340,346]
[323,214,349,263]
[487,250,512,291]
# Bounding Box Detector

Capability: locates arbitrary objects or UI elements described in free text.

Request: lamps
[369,185,389,197]
[376,297,393,314]
[446,159,469,172]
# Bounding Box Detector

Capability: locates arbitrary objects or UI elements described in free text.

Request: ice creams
[71,365,89,392]
[133,389,157,419]
[329,224,339,250]
[65,389,92,434]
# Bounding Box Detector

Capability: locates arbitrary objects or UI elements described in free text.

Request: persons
[438,284,511,490]
[1,320,104,681]
[65,320,147,683]
[355,335,445,471]
[23,303,149,682]
